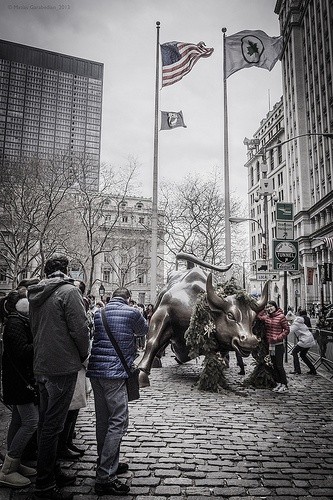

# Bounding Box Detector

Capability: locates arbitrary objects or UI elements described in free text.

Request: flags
[160,40,214,88]
[225,29,283,78]
[160,110,187,130]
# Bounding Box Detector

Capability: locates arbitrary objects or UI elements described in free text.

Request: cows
[133,251,271,389]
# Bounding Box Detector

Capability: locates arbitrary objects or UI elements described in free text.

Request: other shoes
[290,371,301,375]
[22,453,38,467]
[35,486,73,500]
[307,370,317,375]
[277,383,289,393]
[272,382,282,392]
[55,463,76,487]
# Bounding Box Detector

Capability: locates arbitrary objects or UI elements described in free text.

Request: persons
[0,253,154,500]
[226,301,333,394]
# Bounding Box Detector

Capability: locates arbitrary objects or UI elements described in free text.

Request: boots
[15,463,37,477]
[67,435,85,457]
[56,436,81,462]
[0,453,31,487]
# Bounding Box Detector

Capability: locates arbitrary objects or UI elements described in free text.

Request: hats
[285,310,296,320]
[44,256,68,275]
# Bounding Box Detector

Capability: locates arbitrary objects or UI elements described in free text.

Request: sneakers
[96,458,129,477]
[95,479,130,495]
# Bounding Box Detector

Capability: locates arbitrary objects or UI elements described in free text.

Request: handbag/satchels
[125,368,140,402]
[33,386,40,406]
